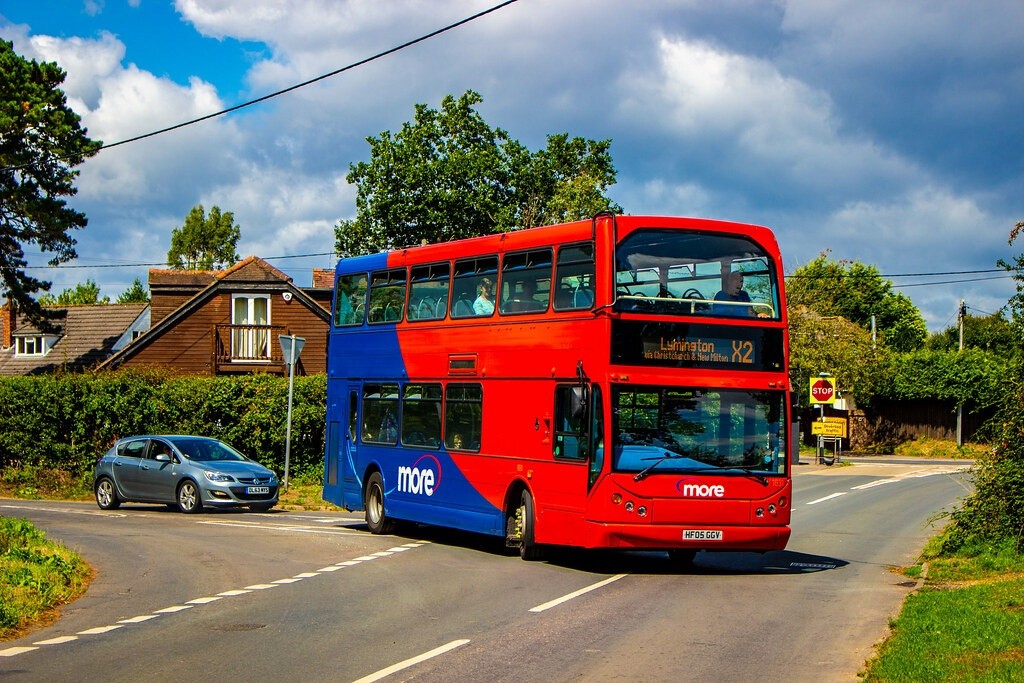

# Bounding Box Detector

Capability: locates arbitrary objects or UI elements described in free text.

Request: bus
[321,210,792,559]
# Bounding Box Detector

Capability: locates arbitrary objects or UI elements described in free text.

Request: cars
[91,434,281,514]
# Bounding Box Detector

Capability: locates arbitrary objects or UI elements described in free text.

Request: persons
[351,278,367,323]
[473,278,502,315]
[713,271,757,317]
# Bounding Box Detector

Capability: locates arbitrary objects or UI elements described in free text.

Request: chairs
[418,295,436,320]
[631,292,654,311]
[408,296,422,319]
[556,283,573,309]
[680,288,711,312]
[654,290,680,310]
[353,304,365,325]
[436,295,449,319]
[454,293,475,316]
[385,300,402,322]
[616,283,631,311]
[573,282,593,308]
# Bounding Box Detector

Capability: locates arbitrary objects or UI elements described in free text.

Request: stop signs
[812,377,835,404]
